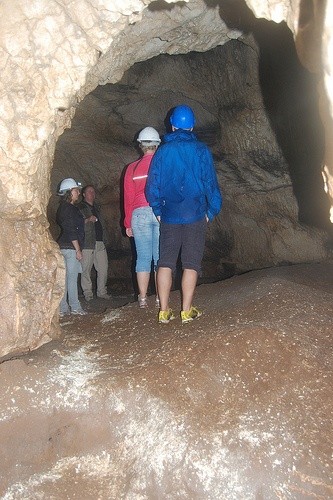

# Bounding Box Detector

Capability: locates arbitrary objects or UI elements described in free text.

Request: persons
[56,178,87,316]
[76,185,112,302]
[123,126,162,309]
[145,105,223,325]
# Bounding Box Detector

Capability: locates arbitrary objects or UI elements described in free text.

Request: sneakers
[158,308,175,323]
[138,295,148,308]
[181,305,202,323]
[155,297,161,308]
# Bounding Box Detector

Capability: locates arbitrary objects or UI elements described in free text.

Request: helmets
[137,126,161,142]
[58,177,78,192]
[170,104,195,129]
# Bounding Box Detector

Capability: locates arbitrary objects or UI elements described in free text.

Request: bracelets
[77,249,81,254]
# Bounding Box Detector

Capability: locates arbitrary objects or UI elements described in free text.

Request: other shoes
[71,309,88,315]
[85,294,93,301]
[97,292,112,299]
